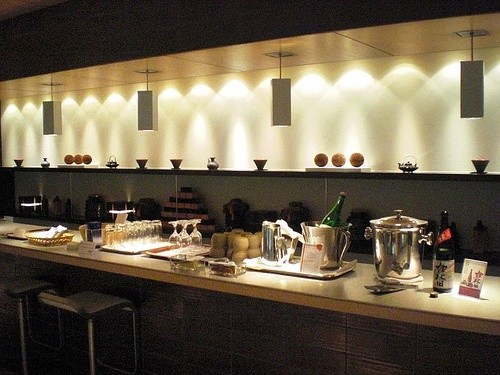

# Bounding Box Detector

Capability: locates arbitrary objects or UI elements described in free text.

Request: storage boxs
[160,186,219,236]
[18,195,42,220]
[208,257,248,279]
[169,254,205,274]
[107,201,137,222]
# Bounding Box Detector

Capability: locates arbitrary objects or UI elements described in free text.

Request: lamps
[454,28,490,119]
[42,81,63,137]
[133,68,163,132]
[263,50,297,128]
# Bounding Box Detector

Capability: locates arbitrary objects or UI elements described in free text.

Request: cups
[105,220,162,248]
[135,159,148,169]
[472,160,490,172]
[253,160,268,170]
[14,159,24,167]
[169,158,183,170]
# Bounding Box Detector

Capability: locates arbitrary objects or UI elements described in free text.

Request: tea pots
[398,156,418,173]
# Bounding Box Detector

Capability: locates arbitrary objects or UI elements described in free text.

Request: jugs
[262,222,287,266]
[207,156,219,170]
[301,220,352,273]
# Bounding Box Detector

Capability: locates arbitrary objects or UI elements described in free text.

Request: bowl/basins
[78,223,107,248]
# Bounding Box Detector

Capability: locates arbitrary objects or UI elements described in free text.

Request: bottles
[41,157,51,167]
[41,195,48,214]
[320,192,348,228]
[432,210,455,292]
[52,195,73,217]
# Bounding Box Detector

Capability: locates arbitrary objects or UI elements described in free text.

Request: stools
[0,276,67,375]
[38,287,140,375]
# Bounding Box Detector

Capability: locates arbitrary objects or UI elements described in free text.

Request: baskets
[25,232,75,246]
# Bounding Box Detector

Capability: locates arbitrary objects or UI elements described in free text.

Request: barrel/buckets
[364,210,433,284]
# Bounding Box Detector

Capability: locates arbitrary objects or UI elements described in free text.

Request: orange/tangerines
[314,153,364,167]
[64,154,92,164]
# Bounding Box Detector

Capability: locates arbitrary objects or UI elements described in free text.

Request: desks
[0,220,500,375]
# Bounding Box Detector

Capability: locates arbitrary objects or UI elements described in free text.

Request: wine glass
[168,218,204,250]
